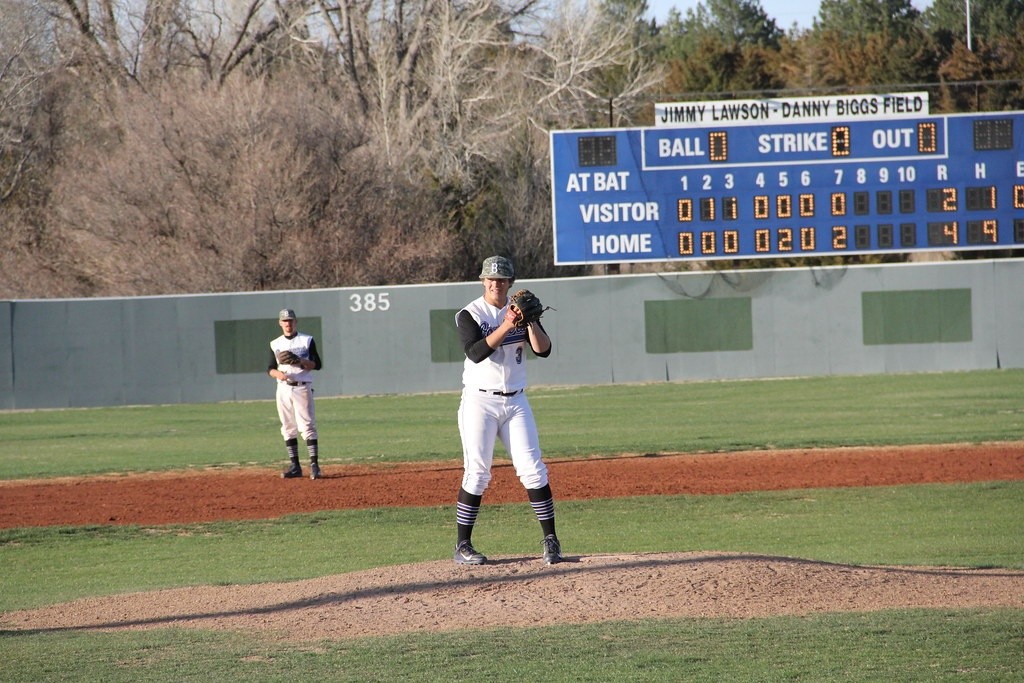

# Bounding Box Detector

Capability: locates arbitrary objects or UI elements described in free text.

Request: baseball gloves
[504,289,543,329]
[277,351,301,366]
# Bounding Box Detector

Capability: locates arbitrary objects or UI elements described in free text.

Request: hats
[479,255,514,278]
[279,308,296,321]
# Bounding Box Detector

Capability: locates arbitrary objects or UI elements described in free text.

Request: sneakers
[281,464,302,478]
[454,540,487,565]
[540,534,561,564]
[310,463,321,480]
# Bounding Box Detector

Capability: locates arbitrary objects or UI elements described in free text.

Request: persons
[452,256,559,565]
[266,309,323,478]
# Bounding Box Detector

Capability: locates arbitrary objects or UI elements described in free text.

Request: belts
[478,388,523,397]
[287,381,307,386]
